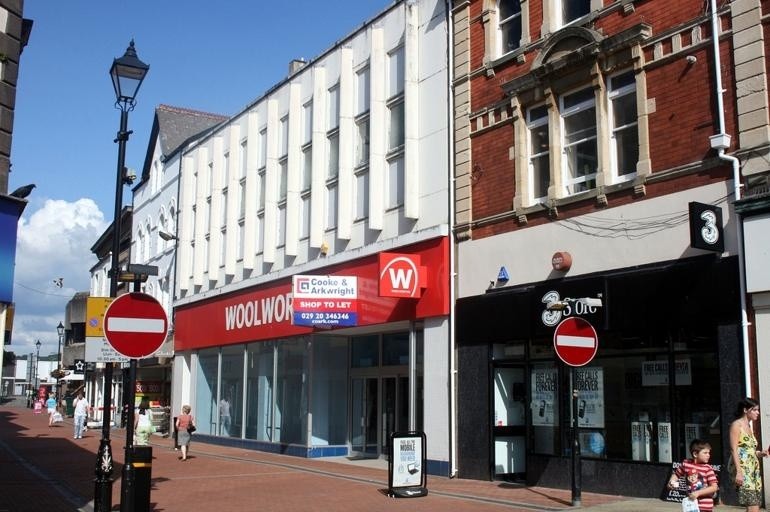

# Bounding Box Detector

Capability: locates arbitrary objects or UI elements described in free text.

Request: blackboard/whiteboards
[388,431,426,489]
[666,461,722,505]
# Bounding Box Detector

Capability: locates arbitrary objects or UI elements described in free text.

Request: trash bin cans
[65,397,74,416]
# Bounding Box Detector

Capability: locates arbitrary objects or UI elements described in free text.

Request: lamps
[547,297,603,311]
[159,230,179,241]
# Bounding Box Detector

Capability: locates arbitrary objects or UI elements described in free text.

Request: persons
[72,390,92,439]
[218,397,232,435]
[666,439,718,512]
[132,398,156,445]
[726,397,770,512]
[46,395,57,427]
[176,404,194,461]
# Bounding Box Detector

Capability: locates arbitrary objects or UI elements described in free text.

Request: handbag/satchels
[187,424,196,432]
[151,426,156,432]
[53,414,63,424]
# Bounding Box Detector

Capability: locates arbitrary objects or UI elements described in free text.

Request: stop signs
[553,316,599,367]
[102,291,169,360]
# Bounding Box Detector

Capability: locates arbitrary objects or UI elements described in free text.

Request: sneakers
[73,436,83,439]
[179,458,185,461]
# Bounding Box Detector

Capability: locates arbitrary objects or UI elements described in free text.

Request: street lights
[94,38,151,508]
[56,321,64,402]
[35,339,41,390]
